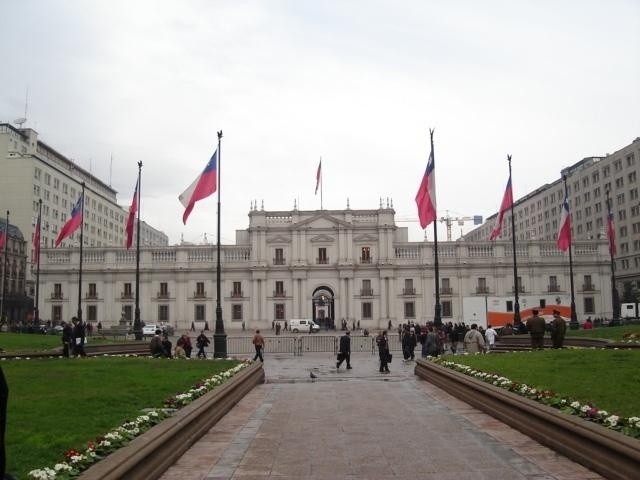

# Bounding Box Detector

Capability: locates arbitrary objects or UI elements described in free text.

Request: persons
[464,324,485,353]
[376,330,391,372]
[398,320,470,362]
[388,319,392,329]
[337,331,352,369]
[0,367,9,480]
[583,319,593,330]
[197,331,211,358]
[253,329,265,362]
[191,321,195,331]
[479,327,486,343]
[141,320,145,328]
[83,321,102,337]
[341,318,355,331]
[502,323,512,335]
[272,320,288,335]
[60,317,87,358]
[550,310,566,348]
[150,329,192,359]
[364,329,368,335]
[309,323,312,334]
[485,325,497,345]
[205,321,209,330]
[526,309,545,349]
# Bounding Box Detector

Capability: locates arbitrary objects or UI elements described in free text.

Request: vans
[290,319,320,335]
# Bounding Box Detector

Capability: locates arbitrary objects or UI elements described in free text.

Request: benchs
[98,329,128,340]
[111,325,133,338]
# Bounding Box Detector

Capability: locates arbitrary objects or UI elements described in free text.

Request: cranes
[396,210,482,241]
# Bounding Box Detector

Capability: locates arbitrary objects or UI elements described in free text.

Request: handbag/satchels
[386,354,392,362]
[337,353,343,359]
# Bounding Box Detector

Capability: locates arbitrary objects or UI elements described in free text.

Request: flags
[315,160,321,194]
[490,176,513,241]
[556,198,571,252]
[125,177,138,250]
[31,214,40,268]
[0,225,6,251]
[607,209,617,256]
[54,192,82,249]
[178,149,217,226]
[415,151,437,229]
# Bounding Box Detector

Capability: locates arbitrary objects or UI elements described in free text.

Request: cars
[141,324,163,336]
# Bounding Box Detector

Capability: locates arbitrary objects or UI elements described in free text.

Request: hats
[532,309,539,312]
[553,309,560,314]
